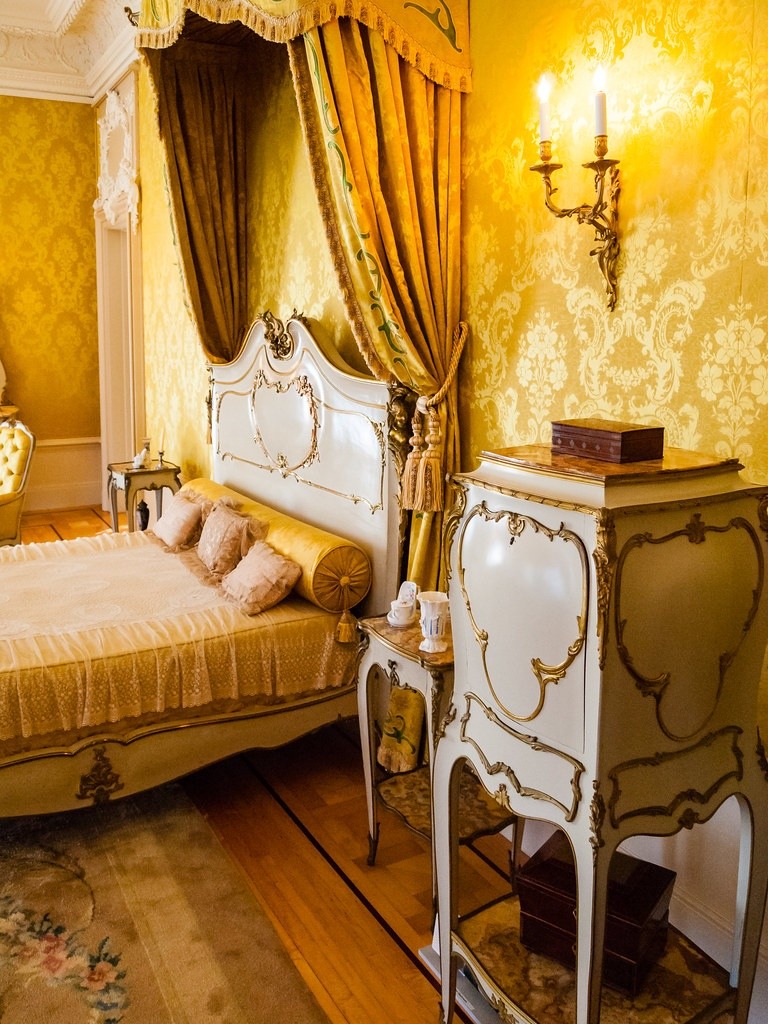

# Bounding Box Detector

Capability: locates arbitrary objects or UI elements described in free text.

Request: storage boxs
[514,829,676,1000]
[550,419,665,464]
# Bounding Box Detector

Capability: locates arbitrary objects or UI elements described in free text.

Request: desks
[106,459,181,533]
[429,439,768,1024]
[355,612,528,934]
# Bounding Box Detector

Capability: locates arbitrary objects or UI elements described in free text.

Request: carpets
[0,782,331,1024]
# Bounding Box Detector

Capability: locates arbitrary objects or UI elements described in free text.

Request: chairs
[0,414,36,547]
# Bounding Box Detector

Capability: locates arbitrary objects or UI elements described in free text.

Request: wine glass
[416,591,449,654]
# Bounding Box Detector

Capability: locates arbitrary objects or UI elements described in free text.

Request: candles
[539,102,551,142]
[159,427,165,451]
[596,91,608,136]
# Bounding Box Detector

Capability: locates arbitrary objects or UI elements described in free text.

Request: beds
[0,310,419,820]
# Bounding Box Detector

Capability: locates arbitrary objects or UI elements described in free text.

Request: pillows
[214,539,302,616]
[179,497,269,585]
[144,486,213,553]
[180,478,372,643]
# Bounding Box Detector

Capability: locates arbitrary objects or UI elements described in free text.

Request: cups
[391,600,413,619]
